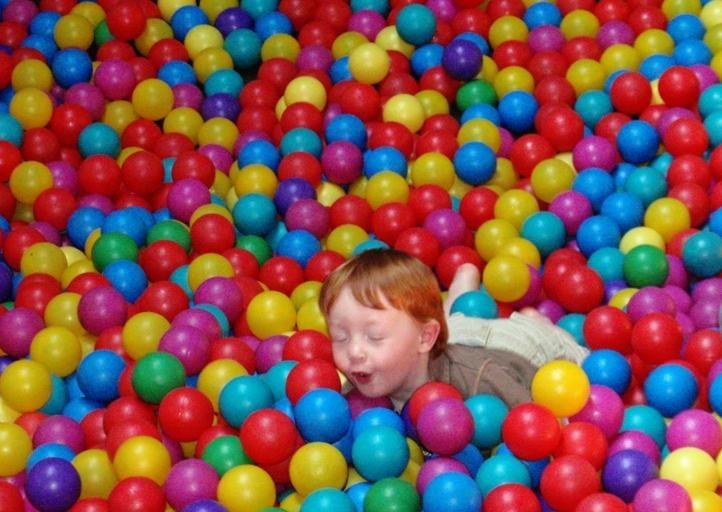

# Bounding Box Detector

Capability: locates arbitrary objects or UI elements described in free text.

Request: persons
[319,247,590,461]
